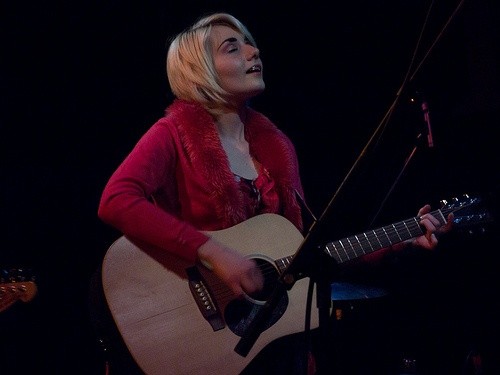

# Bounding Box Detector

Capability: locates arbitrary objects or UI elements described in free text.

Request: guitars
[102,192,484,375]
[1,267,37,311]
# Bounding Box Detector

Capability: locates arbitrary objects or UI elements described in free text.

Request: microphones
[423,103,434,148]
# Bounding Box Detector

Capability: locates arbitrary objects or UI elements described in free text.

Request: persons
[96,13,454,375]
